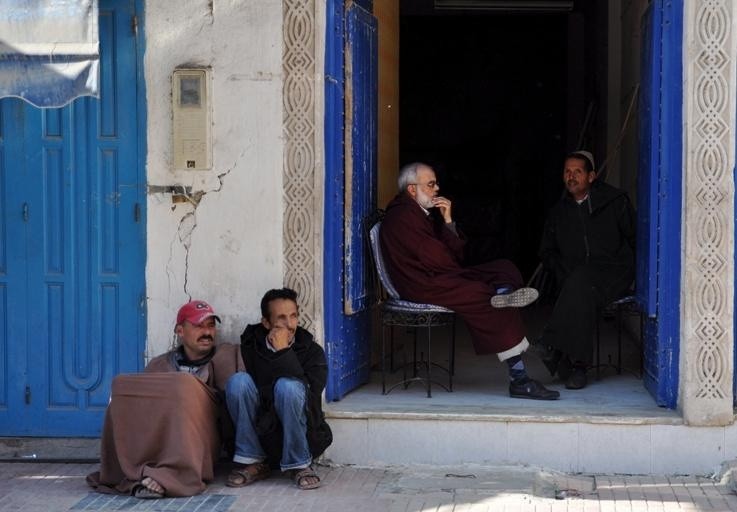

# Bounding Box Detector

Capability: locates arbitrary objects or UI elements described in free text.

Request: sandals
[133,483,165,499]
[289,463,321,490]
[226,462,273,487]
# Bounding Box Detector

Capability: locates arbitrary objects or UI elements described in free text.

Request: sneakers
[566,367,588,389]
[491,287,540,309]
[509,378,560,400]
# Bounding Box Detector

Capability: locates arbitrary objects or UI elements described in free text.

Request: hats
[574,150,595,170]
[177,300,221,326]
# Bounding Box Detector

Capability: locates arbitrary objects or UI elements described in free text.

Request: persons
[223,286,332,490]
[376,162,561,402]
[528,148,637,390]
[83,299,247,500]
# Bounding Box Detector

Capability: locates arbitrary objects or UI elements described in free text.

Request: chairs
[588,211,644,381]
[361,206,457,398]
